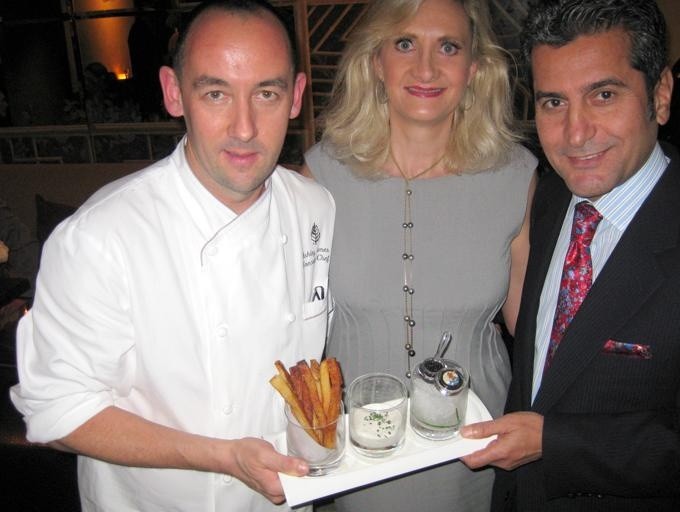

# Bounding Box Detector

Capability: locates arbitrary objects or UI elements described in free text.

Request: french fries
[269,358,343,448]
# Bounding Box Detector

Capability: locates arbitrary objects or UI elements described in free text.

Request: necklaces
[380,110,459,186]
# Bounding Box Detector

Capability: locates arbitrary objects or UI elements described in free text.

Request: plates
[262,388,497,509]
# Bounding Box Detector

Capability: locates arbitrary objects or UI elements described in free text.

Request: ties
[543,200,603,381]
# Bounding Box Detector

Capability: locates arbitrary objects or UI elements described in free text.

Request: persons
[457,0,680,512]
[295,0,541,511]
[8,0,338,512]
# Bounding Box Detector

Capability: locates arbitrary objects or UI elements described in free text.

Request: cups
[346,372,409,458]
[284,398,346,477]
[406,358,470,441]
